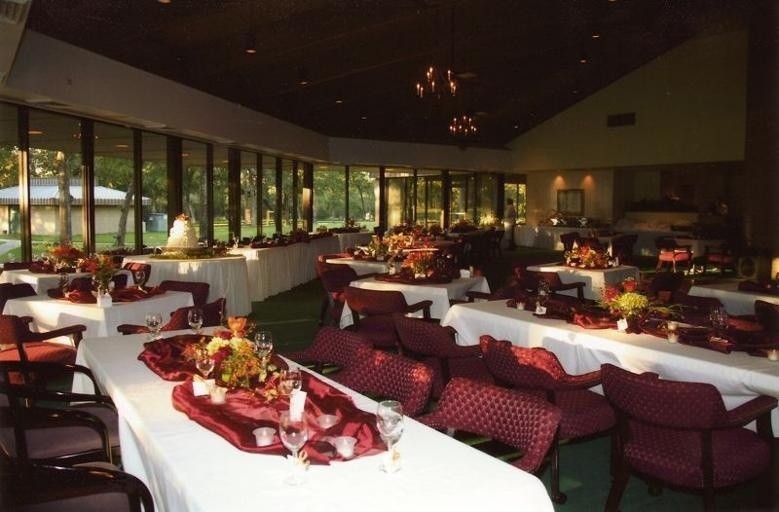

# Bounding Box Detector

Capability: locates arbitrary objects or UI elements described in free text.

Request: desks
[120,254,252,320]
[213,235,339,302]
[334,231,375,251]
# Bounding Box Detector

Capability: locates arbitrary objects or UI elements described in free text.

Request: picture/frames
[557,189,585,217]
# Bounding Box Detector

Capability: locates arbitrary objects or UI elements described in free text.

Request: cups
[377,401,406,474]
[144,311,163,342]
[331,435,359,460]
[193,343,215,380]
[278,366,302,396]
[316,413,341,427]
[253,330,274,368]
[251,425,278,448]
[207,386,229,406]
[187,307,205,334]
[277,410,310,483]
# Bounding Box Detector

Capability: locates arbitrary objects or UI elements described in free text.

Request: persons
[502,198,516,250]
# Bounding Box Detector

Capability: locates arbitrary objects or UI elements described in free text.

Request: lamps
[296,53,313,86]
[588,15,605,41]
[415,62,459,100]
[447,112,480,135]
[243,24,258,52]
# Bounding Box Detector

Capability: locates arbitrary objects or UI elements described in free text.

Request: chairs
[600,363,779,512]
[0,402,155,512]
[341,284,434,325]
[158,280,206,305]
[391,312,478,363]
[655,239,693,274]
[284,324,378,379]
[1,282,40,300]
[704,236,738,277]
[313,250,356,323]
[0,358,125,461]
[512,265,588,299]
[122,261,151,281]
[479,333,620,505]
[322,347,435,416]
[408,376,565,475]
[0,314,88,360]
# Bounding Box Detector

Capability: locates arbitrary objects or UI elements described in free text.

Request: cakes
[167,213,199,247]
